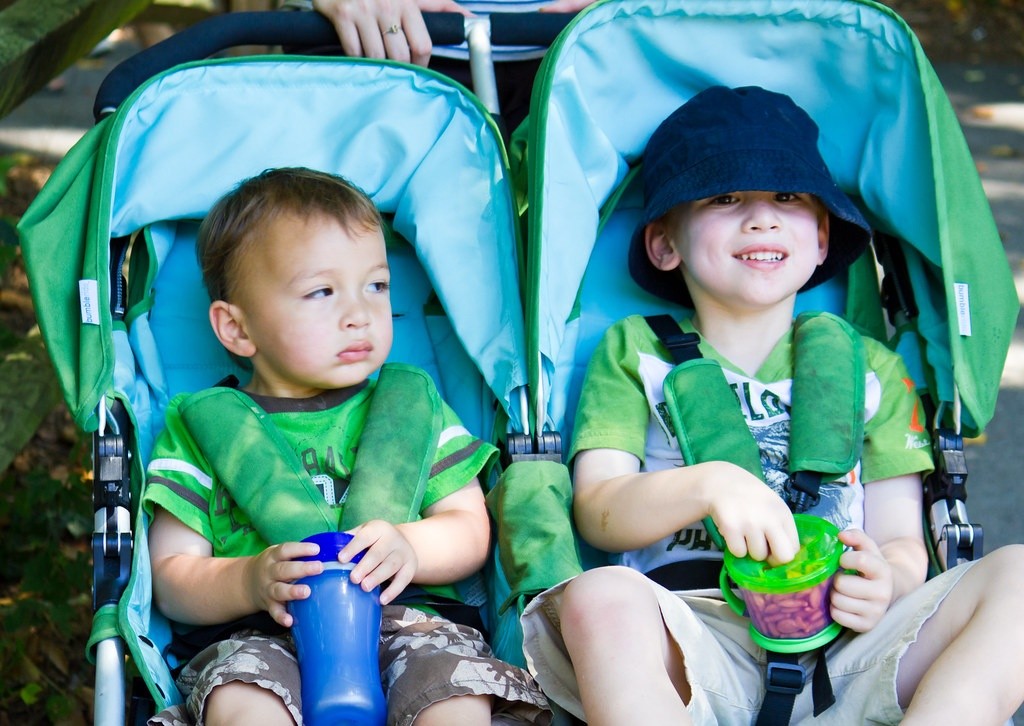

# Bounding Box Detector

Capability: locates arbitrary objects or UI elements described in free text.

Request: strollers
[17,0,1023,726]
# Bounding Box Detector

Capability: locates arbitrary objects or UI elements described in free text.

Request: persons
[519,86,1024,726]
[142,166,554,726]
[282,1,598,114]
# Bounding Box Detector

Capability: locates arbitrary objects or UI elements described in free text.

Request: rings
[384,25,402,36]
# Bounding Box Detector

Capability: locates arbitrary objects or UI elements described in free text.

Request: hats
[628,86,872,309]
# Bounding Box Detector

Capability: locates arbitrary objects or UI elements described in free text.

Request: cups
[719,514,857,653]
[283,531,388,726]
